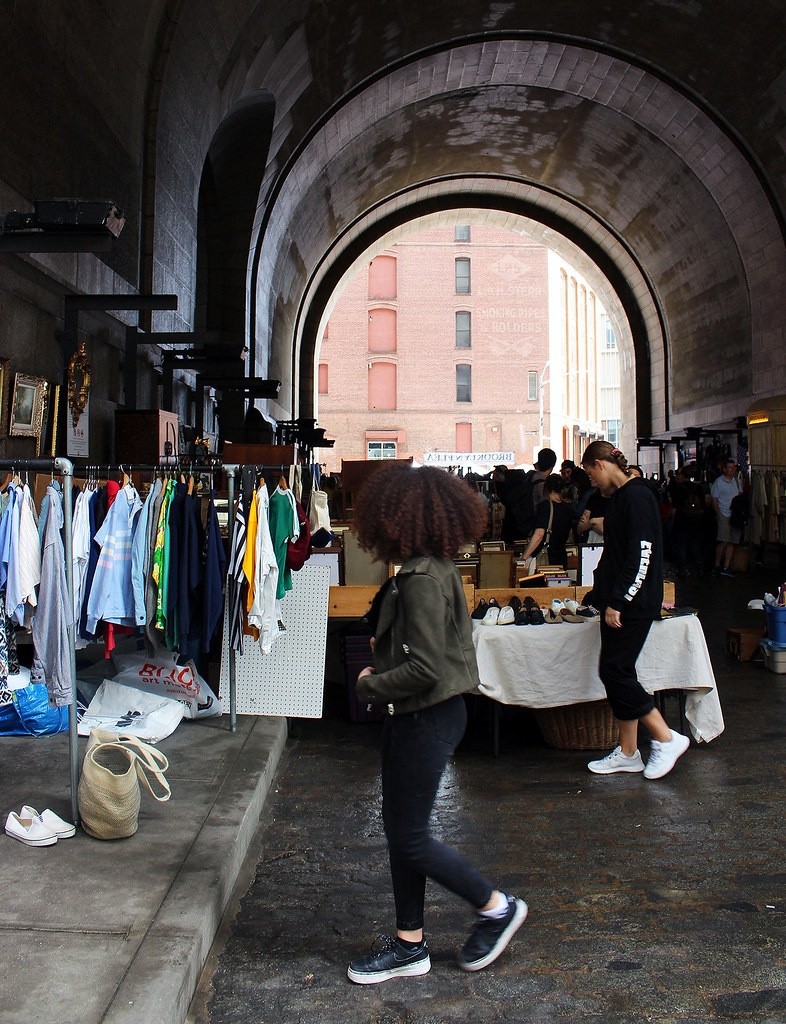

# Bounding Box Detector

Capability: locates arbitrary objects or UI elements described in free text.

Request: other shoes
[712,567,718,577]
[721,570,737,578]
[471,596,600,626]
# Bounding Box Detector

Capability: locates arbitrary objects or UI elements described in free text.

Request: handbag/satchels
[534,548,549,574]
[309,466,335,549]
[287,465,313,571]
[78,729,170,839]
[76,679,185,744]
[0,681,69,739]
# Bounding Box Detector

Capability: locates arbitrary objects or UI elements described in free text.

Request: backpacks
[507,471,546,532]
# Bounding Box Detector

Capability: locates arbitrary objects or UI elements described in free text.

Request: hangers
[0,458,288,496]
[757,464,786,475]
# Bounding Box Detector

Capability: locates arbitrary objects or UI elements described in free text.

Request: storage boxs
[723,627,766,662]
[758,640,786,673]
[762,599,786,649]
[338,626,385,723]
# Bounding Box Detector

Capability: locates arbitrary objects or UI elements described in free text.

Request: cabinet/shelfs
[113,408,178,467]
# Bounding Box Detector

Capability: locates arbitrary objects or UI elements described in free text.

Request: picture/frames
[0,356,13,440]
[35,378,61,458]
[9,372,45,437]
[476,537,576,589]
[68,342,92,428]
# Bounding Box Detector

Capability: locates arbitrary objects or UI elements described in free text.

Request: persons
[520,474,574,571]
[448,448,644,545]
[650,462,718,577]
[320,473,342,520]
[347,464,530,986]
[580,440,691,781]
[710,460,743,578]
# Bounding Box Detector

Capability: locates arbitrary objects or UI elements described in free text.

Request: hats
[560,460,575,472]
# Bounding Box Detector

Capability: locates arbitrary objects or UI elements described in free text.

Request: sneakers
[455,898,529,972]
[348,935,431,984]
[588,746,645,774]
[644,729,691,779]
[20,806,76,838]
[4,811,57,847]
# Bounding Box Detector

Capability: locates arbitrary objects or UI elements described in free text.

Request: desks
[471,612,700,759]
